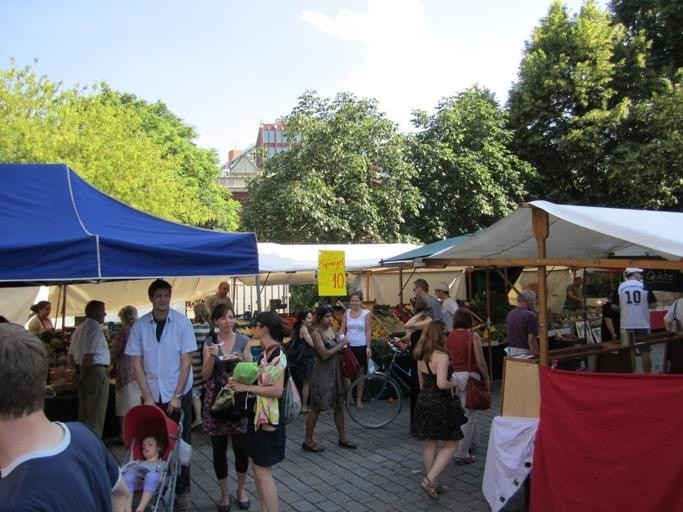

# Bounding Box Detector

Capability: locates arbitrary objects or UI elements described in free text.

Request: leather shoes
[302,441,324,452]
[175,494,191,511]
[219,496,231,512]
[338,439,357,449]
[236,490,250,509]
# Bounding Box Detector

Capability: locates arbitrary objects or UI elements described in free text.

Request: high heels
[191,419,203,430]
[357,400,364,409]
[349,396,354,406]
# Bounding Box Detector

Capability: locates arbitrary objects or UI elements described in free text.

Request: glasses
[254,323,260,328]
[413,287,418,291]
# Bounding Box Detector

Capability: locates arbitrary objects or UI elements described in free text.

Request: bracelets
[366,348,371,351]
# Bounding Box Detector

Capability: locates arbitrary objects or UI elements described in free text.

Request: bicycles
[344,333,410,430]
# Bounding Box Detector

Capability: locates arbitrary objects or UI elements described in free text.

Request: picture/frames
[574,319,594,343]
[592,326,601,343]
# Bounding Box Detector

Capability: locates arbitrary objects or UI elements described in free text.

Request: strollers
[120,403,184,512]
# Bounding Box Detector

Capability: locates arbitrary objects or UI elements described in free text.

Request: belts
[92,364,108,367]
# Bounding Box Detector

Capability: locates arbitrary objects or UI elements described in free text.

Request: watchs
[174,393,184,401]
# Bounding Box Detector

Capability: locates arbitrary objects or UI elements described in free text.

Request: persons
[563,276,585,309]
[611,268,657,374]
[412,320,468,501]
[392,295,433,441]
[228,311,290,512]
[302,308,358,452]
[663,298,683,374]
[339,292,372,410]
[435,281,459,332]
[412,278,442,321]
[207,281,232,312]
[506,289,538,359]
[190,303,214,428]
[28,300,54,335]
[123,279,199,512]
[111,305,142,443]
[124,429,167,512]
[445,308,490,465]
[601,288,621,342]
[201,304,253,512]
[67,300,110,440]
[0,322,131,512]
[290,310,314,414]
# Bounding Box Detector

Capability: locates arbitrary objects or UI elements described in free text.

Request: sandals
[420,477,439,502]
[415,472,448,494]
[455,455,476,465]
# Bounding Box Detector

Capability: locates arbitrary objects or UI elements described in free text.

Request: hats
[433,282,450,293]
[623,268,643,275]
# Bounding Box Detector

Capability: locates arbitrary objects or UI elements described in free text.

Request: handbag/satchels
[210,380,245,418]
[285,376,302,423]
[370,336,394,364]
[465,376,491,411]
[341,343,361,381]
[287,333,310,364]
[442,396,468,431]
